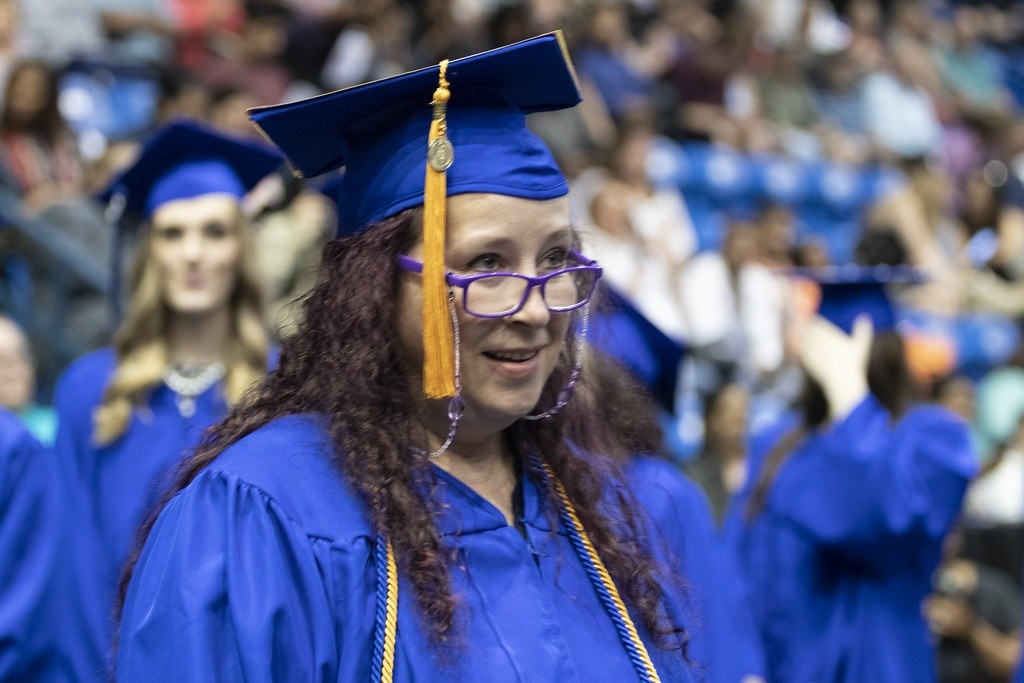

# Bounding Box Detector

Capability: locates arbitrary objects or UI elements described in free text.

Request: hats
[0,194,108,383]
[90,115,286,329]
[784,264,930,338]
[579,266,700,418]
[244,29,583,401]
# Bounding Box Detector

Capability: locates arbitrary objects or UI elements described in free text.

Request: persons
[51,117,284,587]
[0,406,118,683]
[0,0,1024,534]
[110,31,693,683]
[725,264,977,683]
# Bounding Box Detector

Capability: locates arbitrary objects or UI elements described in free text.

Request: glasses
[391,252,602,319]
